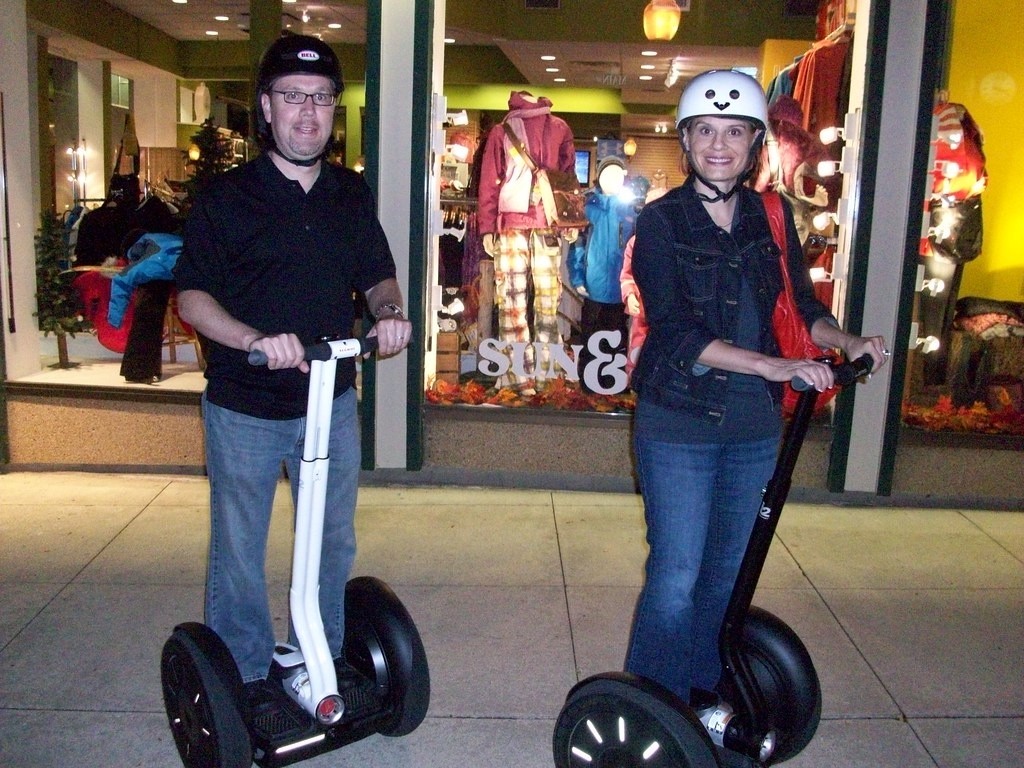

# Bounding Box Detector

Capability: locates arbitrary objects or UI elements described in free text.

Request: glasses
[271,87,338,107]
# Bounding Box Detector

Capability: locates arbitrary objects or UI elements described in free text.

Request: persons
[565,155,637,394]
[621,68,889,707]
[620,187,670,388]
[476,90,576,396]
[175,35,413,710]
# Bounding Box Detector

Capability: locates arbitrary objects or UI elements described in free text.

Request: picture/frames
[574,150,590,186]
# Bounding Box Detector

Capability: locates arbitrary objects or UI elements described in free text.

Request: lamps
[643,0,681,41]
[445,144,468,161]
[808,113,960,355]
[446,110,469,127]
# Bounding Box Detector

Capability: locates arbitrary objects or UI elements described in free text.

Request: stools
[163,288,208,370]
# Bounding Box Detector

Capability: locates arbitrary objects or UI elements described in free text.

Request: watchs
[373,301,405,321]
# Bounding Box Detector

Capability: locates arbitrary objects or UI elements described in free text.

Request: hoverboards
[160,334,430,768]
[552,345,873,767]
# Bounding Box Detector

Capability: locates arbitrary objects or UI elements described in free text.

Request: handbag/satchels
[538,167,591,229]
[761,191,845,419]
[931,191,984,263]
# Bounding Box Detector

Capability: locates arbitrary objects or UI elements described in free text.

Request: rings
[396,336,404,339]
[883,348,891,357]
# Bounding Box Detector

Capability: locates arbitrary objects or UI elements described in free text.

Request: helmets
[259,34,345,96]
[675,69,771,142]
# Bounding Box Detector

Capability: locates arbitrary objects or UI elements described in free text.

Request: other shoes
[242,674,278,712]
[690,686,719,715]
[334,657,357,688]
